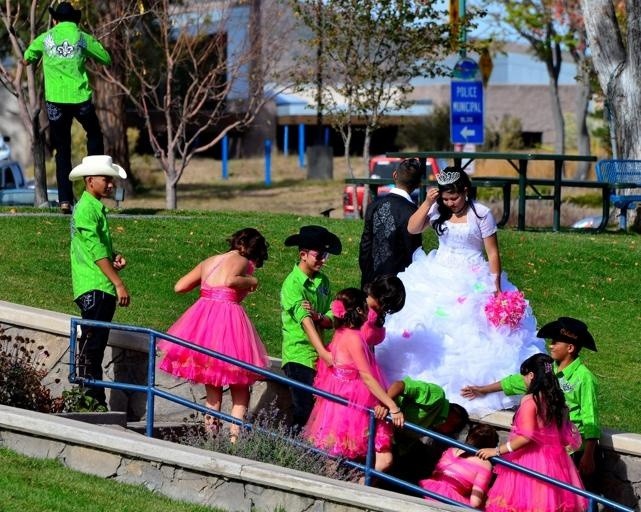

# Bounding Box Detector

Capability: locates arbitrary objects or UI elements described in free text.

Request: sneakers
[58,200,74,214]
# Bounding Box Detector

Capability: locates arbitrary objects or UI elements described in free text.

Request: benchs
[344,175,637,233]
[596,159,640,232]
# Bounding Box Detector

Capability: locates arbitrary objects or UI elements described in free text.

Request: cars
[0,188,59,206]
[1,159,28,191]
[0,131,12,160]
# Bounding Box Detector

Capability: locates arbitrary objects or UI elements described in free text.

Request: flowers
[486,285,533,335]
[330,298,348,319]
[244,258,256,277]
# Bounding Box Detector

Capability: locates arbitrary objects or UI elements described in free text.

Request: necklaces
[452,205,468,216]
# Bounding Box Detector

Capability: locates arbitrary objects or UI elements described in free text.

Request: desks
[385,152,597,231]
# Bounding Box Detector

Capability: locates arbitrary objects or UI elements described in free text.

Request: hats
[284,225,342,256]
[68,154,128,182]
[48,2,82,26]
[536,316,598,353]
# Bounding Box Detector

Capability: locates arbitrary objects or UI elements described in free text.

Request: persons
[374,168,549,418]
[22,1,113,213]
[68,155,131,412]
[280,225,342,424]
[155,228,269,444]
[359,158,424,294]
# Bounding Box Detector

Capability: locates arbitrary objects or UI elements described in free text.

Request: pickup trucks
[338,155,448,222]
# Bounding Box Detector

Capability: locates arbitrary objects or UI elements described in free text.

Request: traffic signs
[449,79,486,145]
